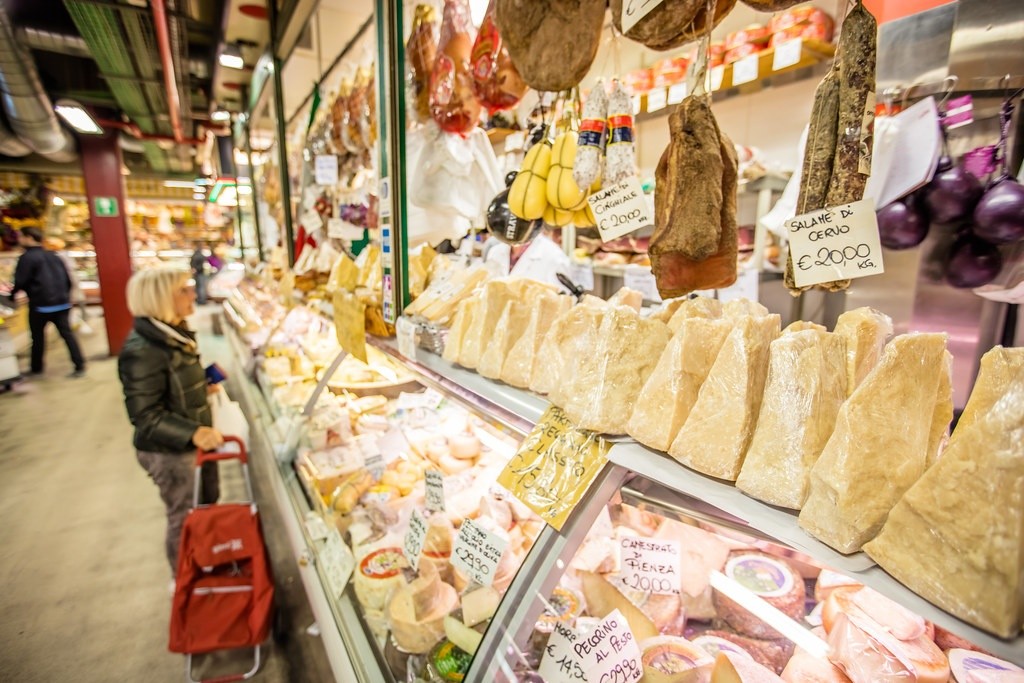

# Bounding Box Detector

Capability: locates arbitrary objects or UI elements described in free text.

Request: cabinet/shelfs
[219,273,1024,680]
[592,166,855,336]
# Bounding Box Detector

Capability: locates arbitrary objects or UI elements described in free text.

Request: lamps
[218,44,244,69]
[54,98,105,135]
[210,106,231,121]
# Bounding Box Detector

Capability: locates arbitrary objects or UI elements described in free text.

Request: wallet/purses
[205,364,226,385]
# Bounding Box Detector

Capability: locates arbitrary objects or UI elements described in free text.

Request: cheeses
[293,245,1024,645]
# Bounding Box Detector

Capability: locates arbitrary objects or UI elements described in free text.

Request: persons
[187,239,207,306]
[3,225,88,379]
[205,243,221,275]
[117,262,226,598]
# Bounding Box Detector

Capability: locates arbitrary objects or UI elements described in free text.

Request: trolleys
[168,435,275,681]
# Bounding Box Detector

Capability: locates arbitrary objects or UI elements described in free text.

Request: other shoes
[72,368,86,377]
[21,370,44,380]
